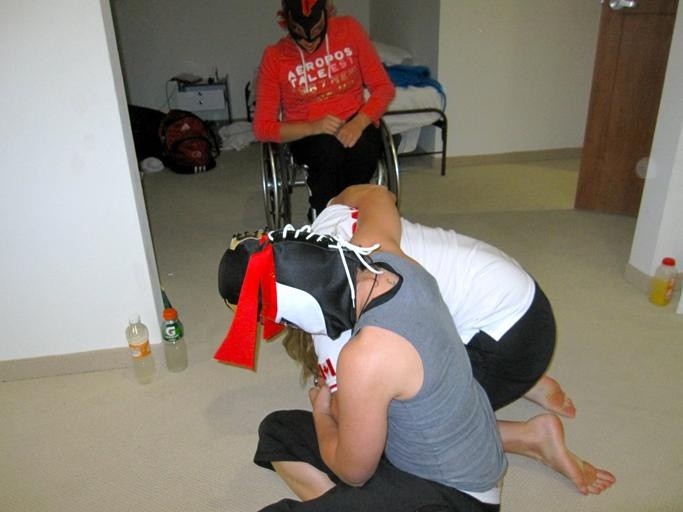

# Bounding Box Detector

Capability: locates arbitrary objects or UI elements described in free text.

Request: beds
[350,63,445,176]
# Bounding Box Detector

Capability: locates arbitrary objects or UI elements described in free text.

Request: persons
[253,1,396,224]
[283,203,615,495]
[220,183,508,512]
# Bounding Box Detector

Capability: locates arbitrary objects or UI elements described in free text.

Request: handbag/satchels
[159,110,220,174]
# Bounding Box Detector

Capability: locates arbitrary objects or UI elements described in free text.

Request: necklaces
[359,270,379,321]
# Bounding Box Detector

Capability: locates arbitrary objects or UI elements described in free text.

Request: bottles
[125,314,164,386]
[162,309,189,374]
[648,258,677,308]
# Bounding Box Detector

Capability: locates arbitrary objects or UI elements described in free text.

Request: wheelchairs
[246,119,417,228]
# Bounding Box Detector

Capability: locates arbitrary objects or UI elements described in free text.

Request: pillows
[375,42,413,66]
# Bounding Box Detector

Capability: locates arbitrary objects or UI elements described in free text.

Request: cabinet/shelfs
[164,73,233,125]
[244,77,283,123]
